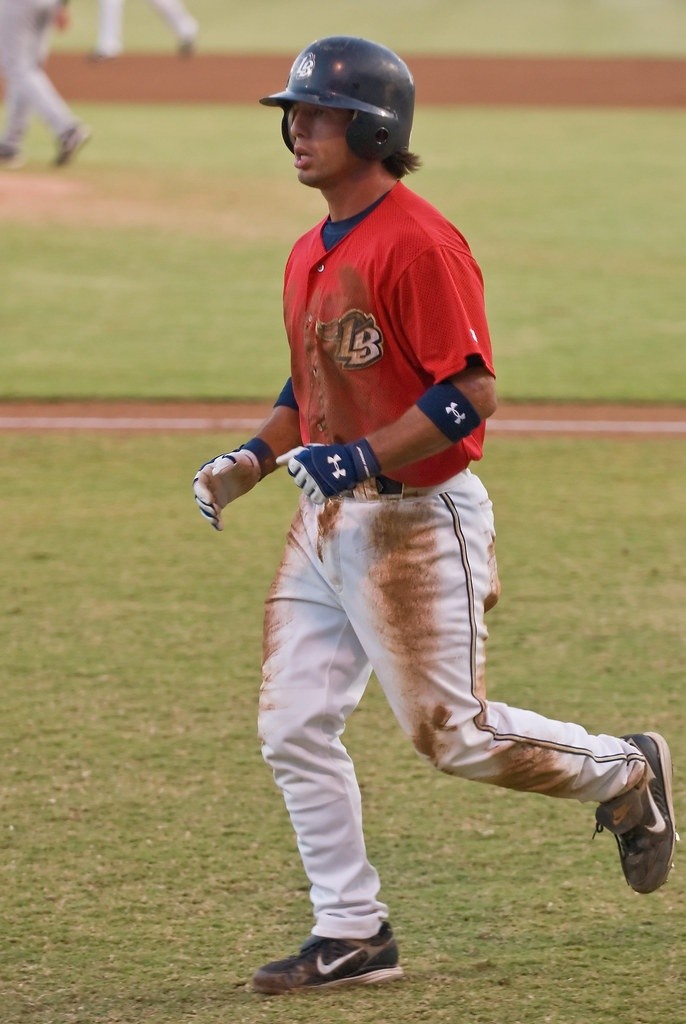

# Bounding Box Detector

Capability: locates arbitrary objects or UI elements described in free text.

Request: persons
[0,0,92,170]
[193,35,673,996]
[95,0,200,59]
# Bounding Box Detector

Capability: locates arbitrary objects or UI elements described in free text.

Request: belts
[374,472,404,495]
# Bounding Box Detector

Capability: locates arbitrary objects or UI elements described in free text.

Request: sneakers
[250,920,405,994]
[595,730,678,895]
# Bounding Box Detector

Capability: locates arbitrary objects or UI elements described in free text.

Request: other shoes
[0,146,29,170]
[53,127,88,171]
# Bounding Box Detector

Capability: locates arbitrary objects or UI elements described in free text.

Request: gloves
[276,438,382,505]
[192,438,280,531]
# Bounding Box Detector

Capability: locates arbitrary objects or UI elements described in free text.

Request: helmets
[258,34,416,161]
